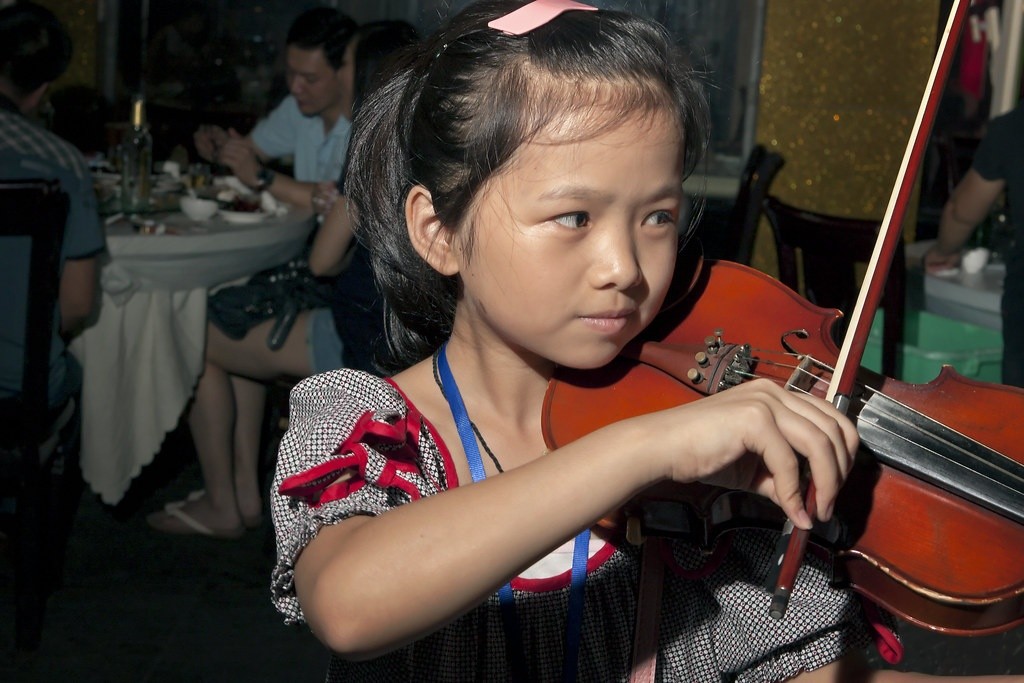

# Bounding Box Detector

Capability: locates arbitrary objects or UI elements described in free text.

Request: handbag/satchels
[206,261,340,347]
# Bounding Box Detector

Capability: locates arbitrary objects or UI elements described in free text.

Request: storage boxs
[918,247,1016,340]
[867,298,1005,390]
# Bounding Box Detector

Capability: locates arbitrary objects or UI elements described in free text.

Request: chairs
[760,192,925,402]
[0,175,83,502]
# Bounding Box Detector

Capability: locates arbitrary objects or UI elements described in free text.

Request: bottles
[119,93,152,215]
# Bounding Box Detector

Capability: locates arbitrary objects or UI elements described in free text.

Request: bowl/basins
[178,197,218,222]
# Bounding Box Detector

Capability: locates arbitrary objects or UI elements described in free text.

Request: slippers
[148,484,265,541]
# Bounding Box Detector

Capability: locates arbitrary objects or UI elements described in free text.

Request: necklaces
[431,343,504,475]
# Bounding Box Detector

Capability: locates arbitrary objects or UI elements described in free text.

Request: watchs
[250,168,275,190]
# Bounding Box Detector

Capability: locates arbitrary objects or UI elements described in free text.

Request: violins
[540,256,1024,636]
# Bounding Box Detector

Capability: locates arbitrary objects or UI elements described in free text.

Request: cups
[188,163,210,190]
[137,195,168,235]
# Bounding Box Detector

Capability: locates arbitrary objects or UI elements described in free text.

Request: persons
[922,105,1024,390]
[142,6,423,543]
[0,0,106,494]
[269,0,1024,683]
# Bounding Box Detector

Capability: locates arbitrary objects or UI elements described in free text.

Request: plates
[219,210,270,225]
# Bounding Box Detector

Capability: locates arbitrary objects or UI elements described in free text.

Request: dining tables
[62,173,315,510]
